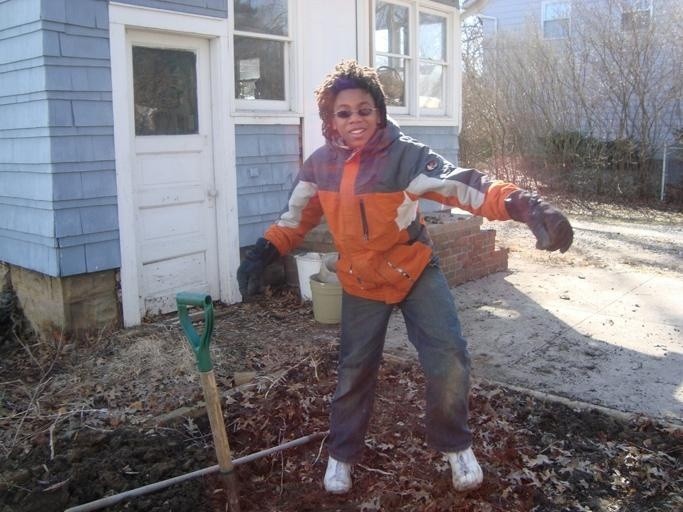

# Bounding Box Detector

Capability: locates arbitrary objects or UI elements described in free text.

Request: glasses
[332,108,376,117]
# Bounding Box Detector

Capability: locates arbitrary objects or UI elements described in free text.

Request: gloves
[504,189,573,253]
[237,237,279,295]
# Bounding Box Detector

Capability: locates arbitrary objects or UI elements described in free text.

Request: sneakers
[446,446,482,491]
[324,456,352,493]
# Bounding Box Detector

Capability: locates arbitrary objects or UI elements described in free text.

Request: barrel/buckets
[309,274,343,324]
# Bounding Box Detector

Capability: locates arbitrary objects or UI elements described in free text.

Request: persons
[236,58,576,496]
